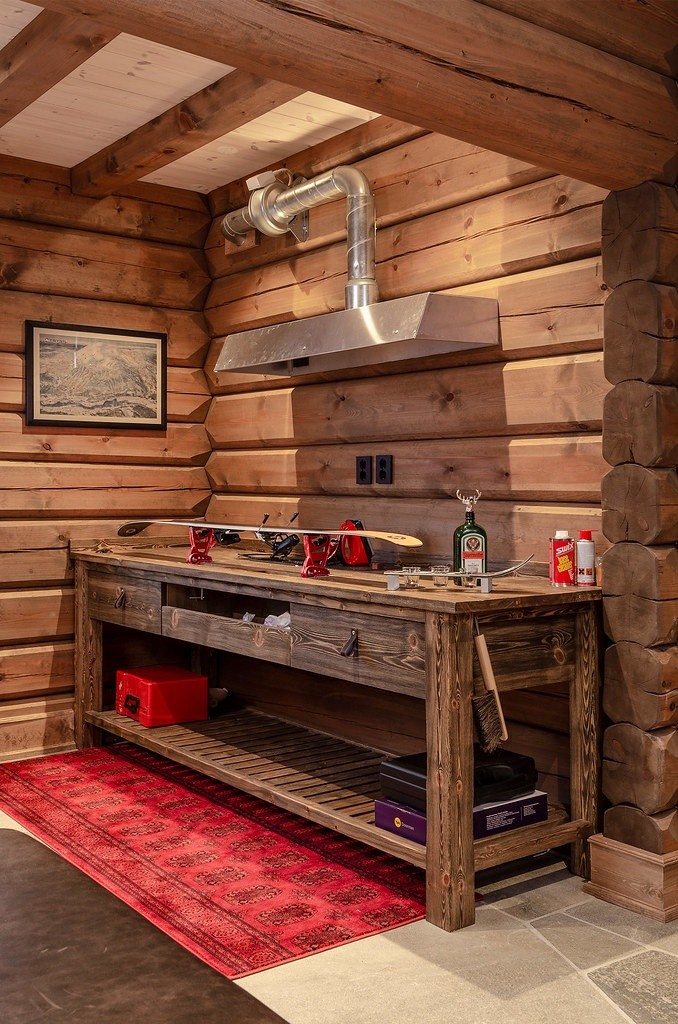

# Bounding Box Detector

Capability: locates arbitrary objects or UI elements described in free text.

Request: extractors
[213,291,500,380]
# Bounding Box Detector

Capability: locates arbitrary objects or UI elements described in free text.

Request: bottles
[453,511,488,588]
[549,531,575,586]
[576,529,596,586]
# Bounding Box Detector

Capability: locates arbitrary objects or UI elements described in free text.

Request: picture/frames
[25,320,167,431]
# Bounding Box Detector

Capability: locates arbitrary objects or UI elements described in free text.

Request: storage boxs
[379,742,538,813]
[374,788,548,847]
[115,663,208,728]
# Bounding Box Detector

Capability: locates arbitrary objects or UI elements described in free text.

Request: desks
[68,534,603,932]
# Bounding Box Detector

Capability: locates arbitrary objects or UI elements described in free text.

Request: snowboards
[116,514,426,580]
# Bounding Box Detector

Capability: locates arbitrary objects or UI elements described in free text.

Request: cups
[430,566,449,587]
[402,567,422,589]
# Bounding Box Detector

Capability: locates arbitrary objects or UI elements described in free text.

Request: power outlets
[356,456,372,484]
[376,455,392,484]
